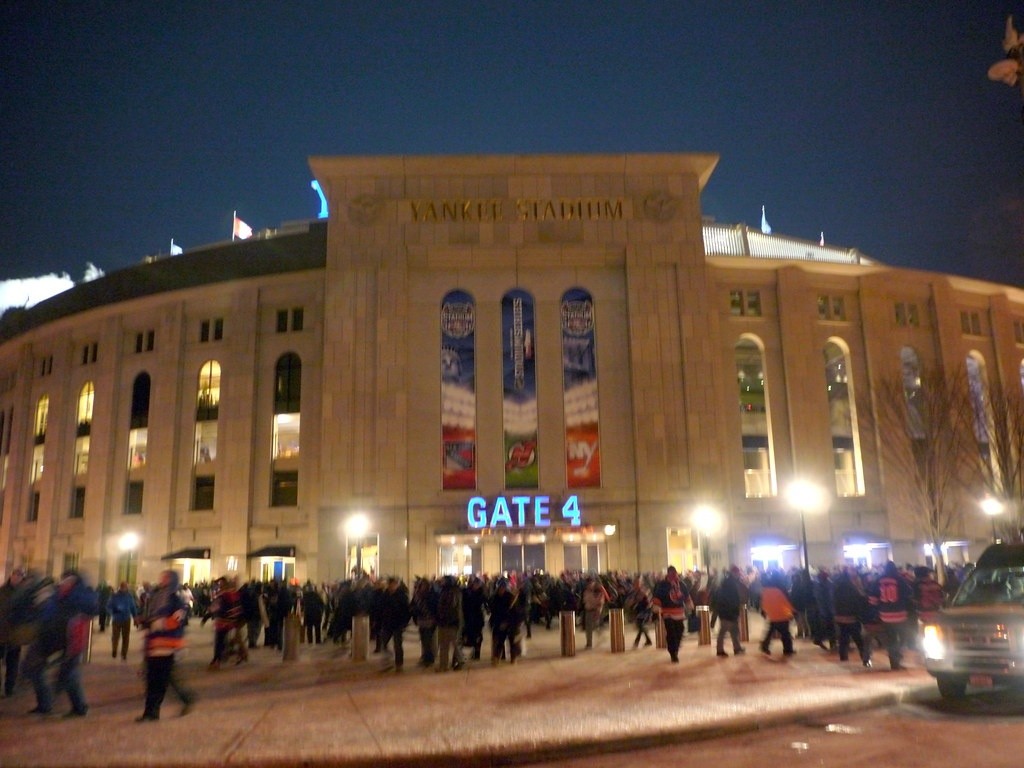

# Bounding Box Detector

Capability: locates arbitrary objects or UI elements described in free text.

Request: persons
[135,570,199,725]
[0,561,1024,722]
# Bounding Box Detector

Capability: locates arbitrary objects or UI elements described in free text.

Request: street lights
[118,534,140,586]
[346,514,368,584]
[789,477,817,579]
[692,502,719,581]
[981,494,1002,545]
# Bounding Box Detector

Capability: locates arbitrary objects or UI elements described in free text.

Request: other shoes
[63,708,86,719]
[716,650,727,656]
[783,650,796,656]
[29,702,51,715]
[181,695,196,715]
[671,655,679,662]
[734,648,745,655]
[135,711,160,722]
[452,660,463,670]
[760,643,771,655]
[891,664,905,670]
[435,666,449,673]
[863,660,871,667]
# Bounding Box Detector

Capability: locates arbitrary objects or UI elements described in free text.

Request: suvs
[919,541,1024,698]
